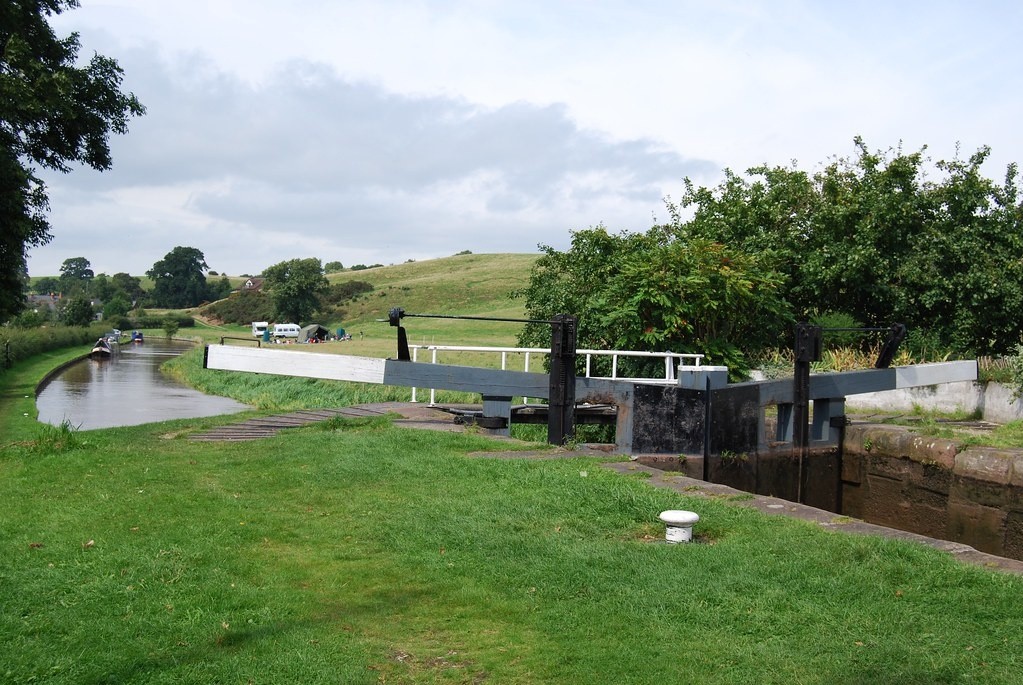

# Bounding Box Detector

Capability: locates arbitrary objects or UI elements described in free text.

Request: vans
[273,324,302,338]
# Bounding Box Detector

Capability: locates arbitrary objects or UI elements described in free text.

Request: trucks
[250,321,268,338]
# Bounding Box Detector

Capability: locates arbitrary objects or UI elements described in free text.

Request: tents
[297,323,331,344]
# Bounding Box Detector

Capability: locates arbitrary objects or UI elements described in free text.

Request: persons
[272,331,363,344]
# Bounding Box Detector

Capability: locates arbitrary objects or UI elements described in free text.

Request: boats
[92,337,112,359]
[132,330,147,346]
